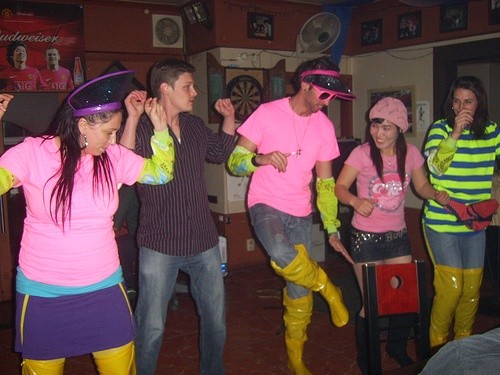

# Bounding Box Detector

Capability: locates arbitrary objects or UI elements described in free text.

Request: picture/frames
[361,19,381,47]
[397,9,421,40]
[488,0,500,26]
[368,85,416,138]
[438,1,468,33]
[246,13,273,41]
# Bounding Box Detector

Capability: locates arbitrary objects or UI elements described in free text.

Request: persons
[263,19,271,37]
[363,24,378,43]
[0,41,49,92]
[0,71,174,375]
[251,21,264,36]
[227,59,355,375]
[423,75,500,347]
[334,97,450,375]
[399,18,417,38]
[418,327,500,375]
[37,47,74,90]
[444,14,464,27]
[116,58,235,375]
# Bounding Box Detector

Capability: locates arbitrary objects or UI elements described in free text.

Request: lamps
[181,0,212,30]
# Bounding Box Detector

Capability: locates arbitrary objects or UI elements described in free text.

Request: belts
[351,227,407,252]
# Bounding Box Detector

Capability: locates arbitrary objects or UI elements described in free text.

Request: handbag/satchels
[443,199,500,230]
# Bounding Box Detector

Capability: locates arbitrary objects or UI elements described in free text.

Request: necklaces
[293,96,311,159]
[382,153,392,166]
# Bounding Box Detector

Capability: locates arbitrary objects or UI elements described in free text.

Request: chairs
[363,256,433,375]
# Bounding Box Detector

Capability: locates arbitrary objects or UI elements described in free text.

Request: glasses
[311,84,337,102]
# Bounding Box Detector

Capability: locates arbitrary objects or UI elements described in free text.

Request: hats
[300,61,355,101]
[369,97,409,134]
[69,69,138,117]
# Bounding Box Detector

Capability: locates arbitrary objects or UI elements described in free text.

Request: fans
[296,11,341,58]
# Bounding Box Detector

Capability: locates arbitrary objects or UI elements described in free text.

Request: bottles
[73,57,84,89]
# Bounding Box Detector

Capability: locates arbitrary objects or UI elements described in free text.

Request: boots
[429,264,463,347]
[91,341,137,375]
[270,243,349,328]
[281,285,312,375]
[454,267,484,341]
[385,312,417,365]
[20,356,65,375]
[356,312,384,375]
